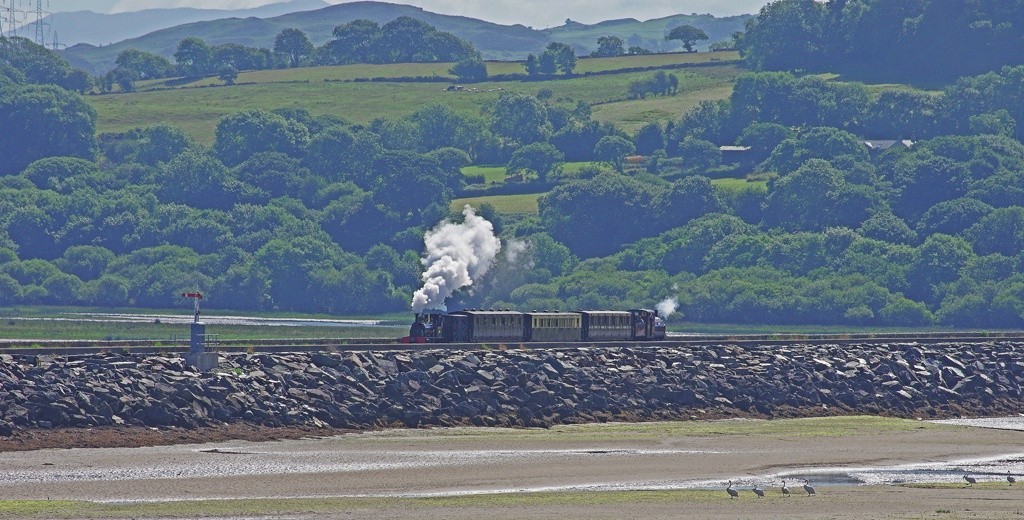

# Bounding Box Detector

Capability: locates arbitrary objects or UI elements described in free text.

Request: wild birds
[781,480,791,497]
[803,480,816,497]
[1007,470,1016,486]
[725,480,739,499]
[963,474,978,487]
[752,485,765,498]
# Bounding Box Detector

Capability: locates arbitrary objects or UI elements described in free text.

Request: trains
[409,307,666,344]
